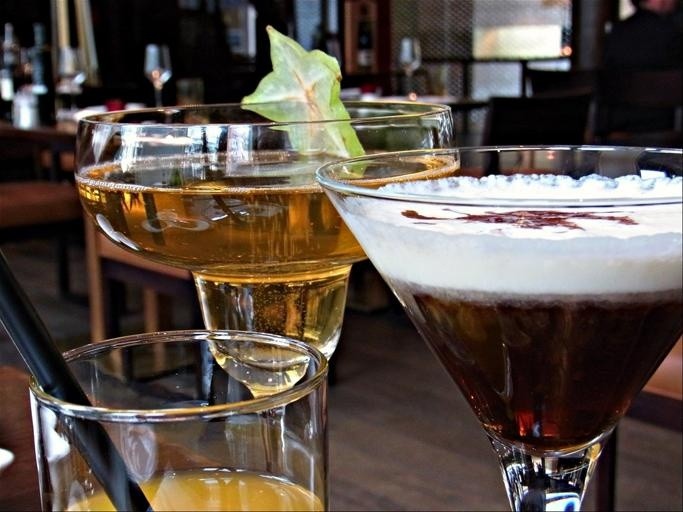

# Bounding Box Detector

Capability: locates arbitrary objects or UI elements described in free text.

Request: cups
[27,329,327,512]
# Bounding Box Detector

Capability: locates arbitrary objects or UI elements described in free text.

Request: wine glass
[314,145,682,511]
[76,97,459,480]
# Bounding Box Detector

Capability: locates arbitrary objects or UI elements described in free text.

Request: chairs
[480,59,602,146]
[0,123,129,319]
